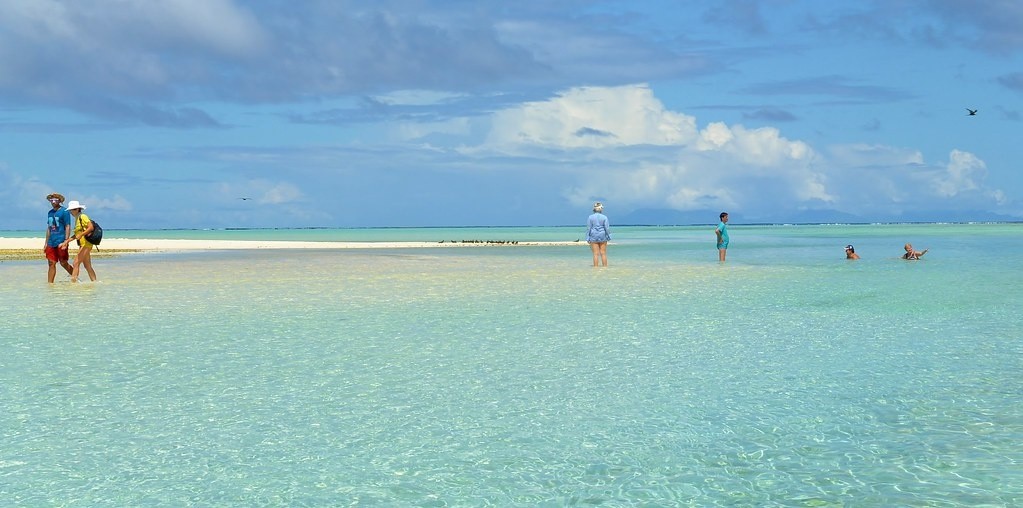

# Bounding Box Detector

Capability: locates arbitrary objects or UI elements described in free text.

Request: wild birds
[963,108,978,116]
[573,238,580,243]
[439,239,519,244]
[236,198,253,201]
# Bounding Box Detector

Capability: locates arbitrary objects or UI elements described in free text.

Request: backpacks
[79,213,102,245]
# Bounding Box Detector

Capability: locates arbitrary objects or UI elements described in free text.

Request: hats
[844,245,853,250]
[46,192,65,203]
[64,201,86,211]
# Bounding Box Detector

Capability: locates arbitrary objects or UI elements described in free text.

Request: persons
[846,245,860,259]
[43,192,97,283]
[902,243,929,260]
[586,202,612,266]
[714,212,729,262]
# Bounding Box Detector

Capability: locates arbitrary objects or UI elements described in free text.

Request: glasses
[846,250,848,252]
[50,199,59,203]
